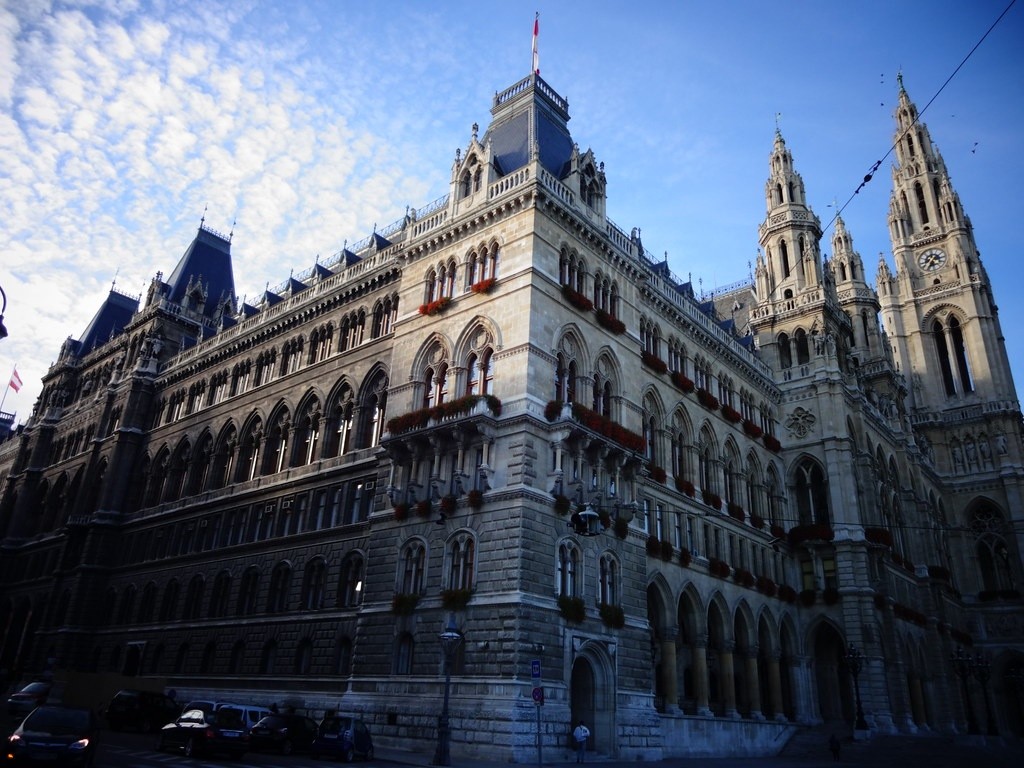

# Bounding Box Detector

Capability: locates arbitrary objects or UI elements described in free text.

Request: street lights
[971,652,1001,736]
[949,643,981,736]
[1006,666,1024,739]
[842,639,870,731]
[429,627,464,766]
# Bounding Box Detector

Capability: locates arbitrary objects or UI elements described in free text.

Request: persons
[828,735,841,763]
[573,720,590,764]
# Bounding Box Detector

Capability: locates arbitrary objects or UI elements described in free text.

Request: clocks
[918,248,946,271]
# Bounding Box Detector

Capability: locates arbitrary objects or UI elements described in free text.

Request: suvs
[105,688,180,734]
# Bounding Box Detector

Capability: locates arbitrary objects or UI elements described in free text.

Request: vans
[181,700,276,728]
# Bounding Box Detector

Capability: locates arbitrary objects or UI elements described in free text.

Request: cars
[7,681,57,724]
[249,714,318,755]
[154,708,250,761]
[310,716,376,761]
[1,706,102,768]
